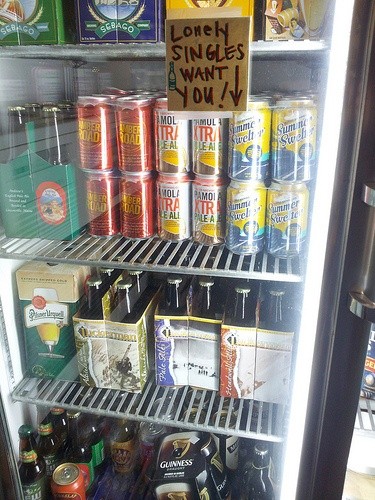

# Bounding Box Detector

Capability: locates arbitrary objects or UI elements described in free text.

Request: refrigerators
[0,0,375,500]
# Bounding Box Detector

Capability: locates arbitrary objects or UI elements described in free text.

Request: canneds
[51,385,243,500]
[75,85,321,260]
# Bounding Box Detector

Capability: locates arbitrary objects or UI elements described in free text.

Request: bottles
[84,241,302,332]
[17,387,282,500]
[7,100,77,166]
